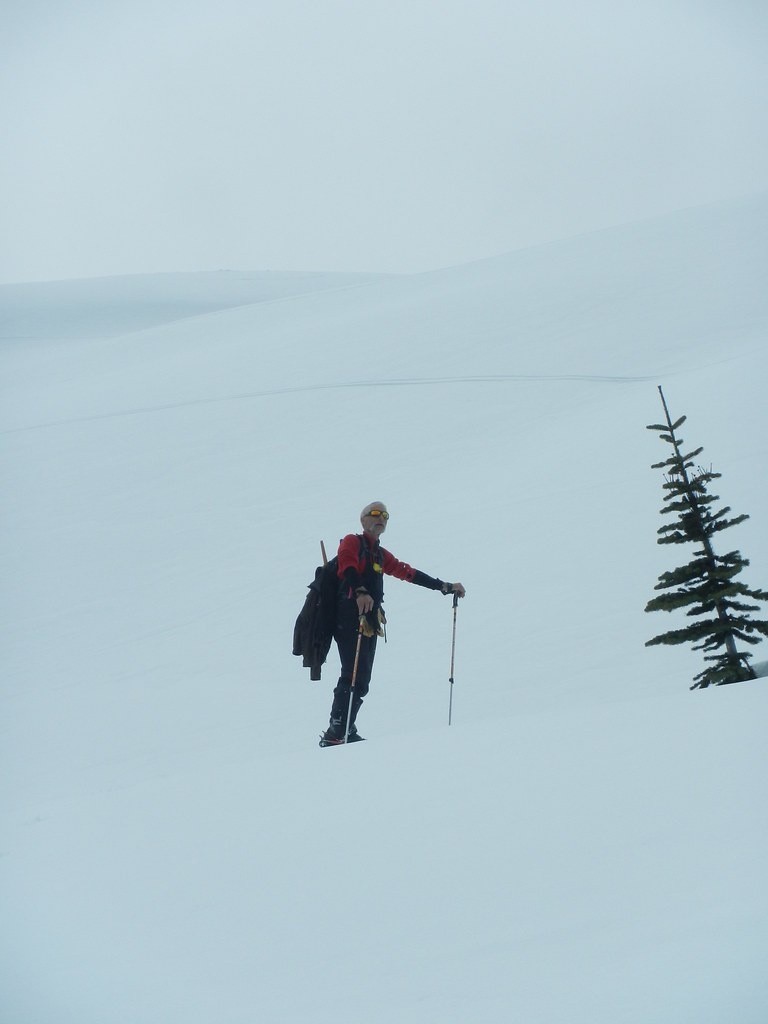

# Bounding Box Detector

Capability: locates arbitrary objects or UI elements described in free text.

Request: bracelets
[448,583,453,593]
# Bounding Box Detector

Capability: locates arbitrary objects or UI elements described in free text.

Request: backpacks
[316,534,383,632]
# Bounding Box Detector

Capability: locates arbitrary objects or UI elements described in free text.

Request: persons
[318,501,466,748]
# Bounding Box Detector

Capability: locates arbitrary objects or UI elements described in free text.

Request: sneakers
[319,727,365,747]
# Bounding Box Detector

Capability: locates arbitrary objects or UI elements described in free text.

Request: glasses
[364,510,389,520]
